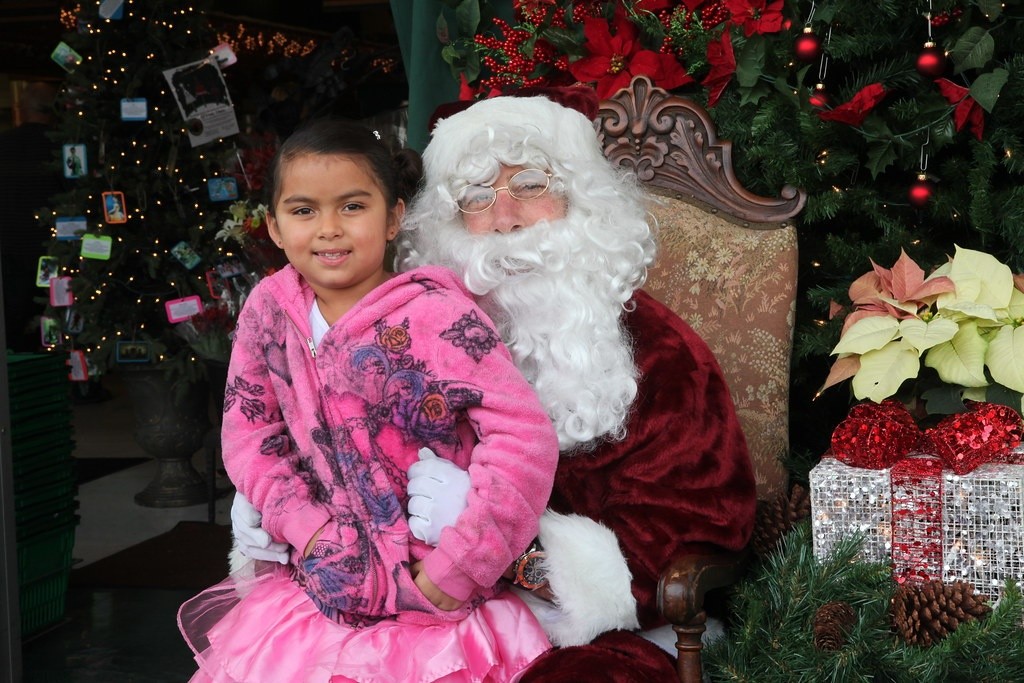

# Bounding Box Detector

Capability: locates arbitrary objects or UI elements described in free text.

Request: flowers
[432,0,1005,182]
[190,142,276,340]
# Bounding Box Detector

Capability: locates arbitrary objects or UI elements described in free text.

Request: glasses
[456,167,553,215]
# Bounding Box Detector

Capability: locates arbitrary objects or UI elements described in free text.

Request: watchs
[513,537,548,591]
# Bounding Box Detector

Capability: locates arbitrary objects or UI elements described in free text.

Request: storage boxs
[809,456,1024,608]
[0,351,84,683]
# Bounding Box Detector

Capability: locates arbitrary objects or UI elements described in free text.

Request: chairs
[593,76,819,683]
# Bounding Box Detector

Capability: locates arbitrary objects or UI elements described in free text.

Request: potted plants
[26,0,280,508]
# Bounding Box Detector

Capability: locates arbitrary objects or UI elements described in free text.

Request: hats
[427,85,600,141]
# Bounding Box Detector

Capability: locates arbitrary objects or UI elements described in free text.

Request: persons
[109,196,124,222]
[39,265,52,284]
[232,84,759,683]
[178,119,558,683]
[67,148,84,175]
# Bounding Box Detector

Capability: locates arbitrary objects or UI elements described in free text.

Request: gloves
[407,446,473,548]
[231,490,290,564]
